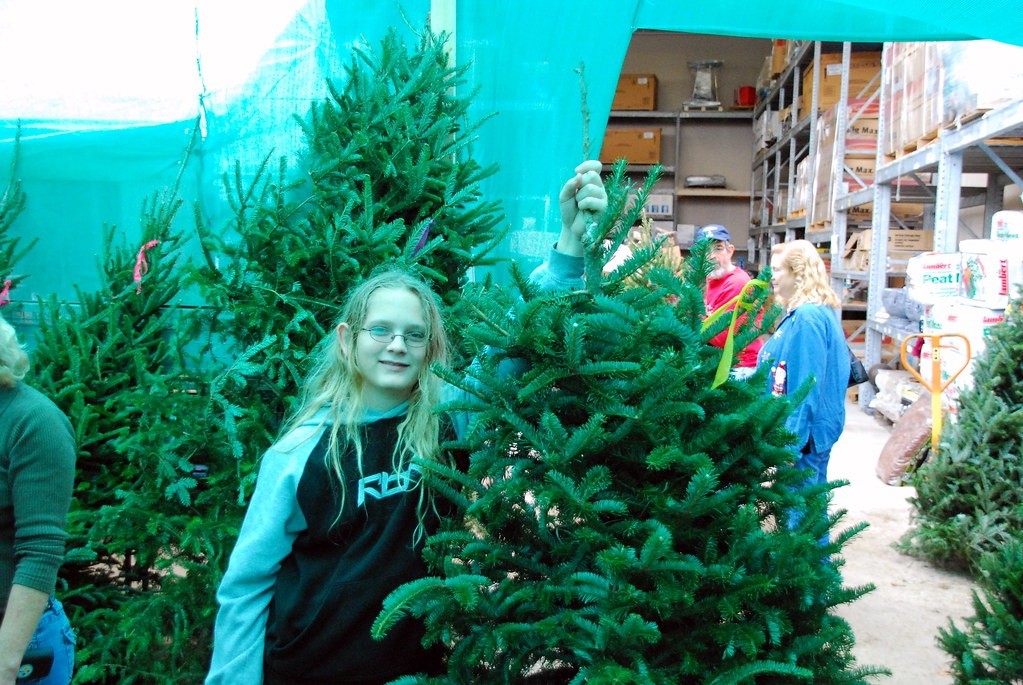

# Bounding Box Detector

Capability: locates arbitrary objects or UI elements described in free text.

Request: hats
[692,224,730,242]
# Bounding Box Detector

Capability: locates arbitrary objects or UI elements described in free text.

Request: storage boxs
[622,194,674,216]
[675,223,696,246]
[753,42,1022,402]
[599,127,661,165]
[611,73,658,111]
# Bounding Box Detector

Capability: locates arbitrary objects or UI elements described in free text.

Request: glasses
[357,326,428,347]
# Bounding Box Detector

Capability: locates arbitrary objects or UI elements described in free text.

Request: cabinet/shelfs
[747,39,1022,424]
[594,109,759,269]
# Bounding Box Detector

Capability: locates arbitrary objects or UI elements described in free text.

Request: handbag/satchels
[845,344,870,390]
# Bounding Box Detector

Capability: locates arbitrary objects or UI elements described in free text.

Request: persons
[757,238,851,562]
[0,314,77,685]
[696,224,765,381]
[205,161,608,685]
